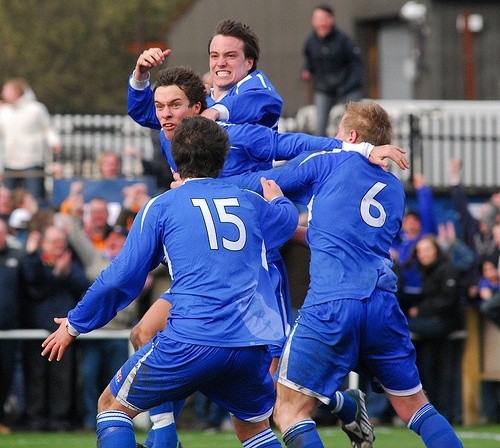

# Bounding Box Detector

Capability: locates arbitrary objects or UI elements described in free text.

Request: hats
[8,208,33,228]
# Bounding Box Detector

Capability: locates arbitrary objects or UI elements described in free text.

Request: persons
[300,4,368,138]
[0,18,500,448]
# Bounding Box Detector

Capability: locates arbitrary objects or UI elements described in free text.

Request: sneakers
[342,389,375,448]
